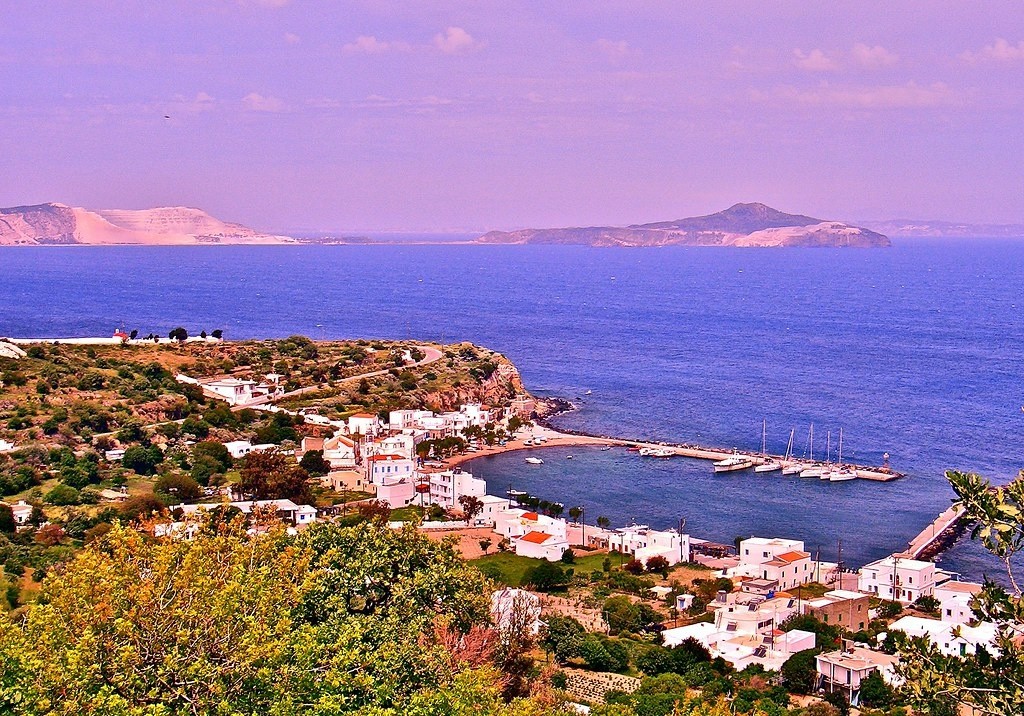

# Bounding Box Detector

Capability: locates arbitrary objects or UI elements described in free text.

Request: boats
[507,489,526,496]
[712,446,753,473]
[599,444,677,458]
[566,455,572,459]
[524,457,545,464]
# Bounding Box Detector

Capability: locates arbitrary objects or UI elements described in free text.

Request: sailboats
[751,420,858,481]
